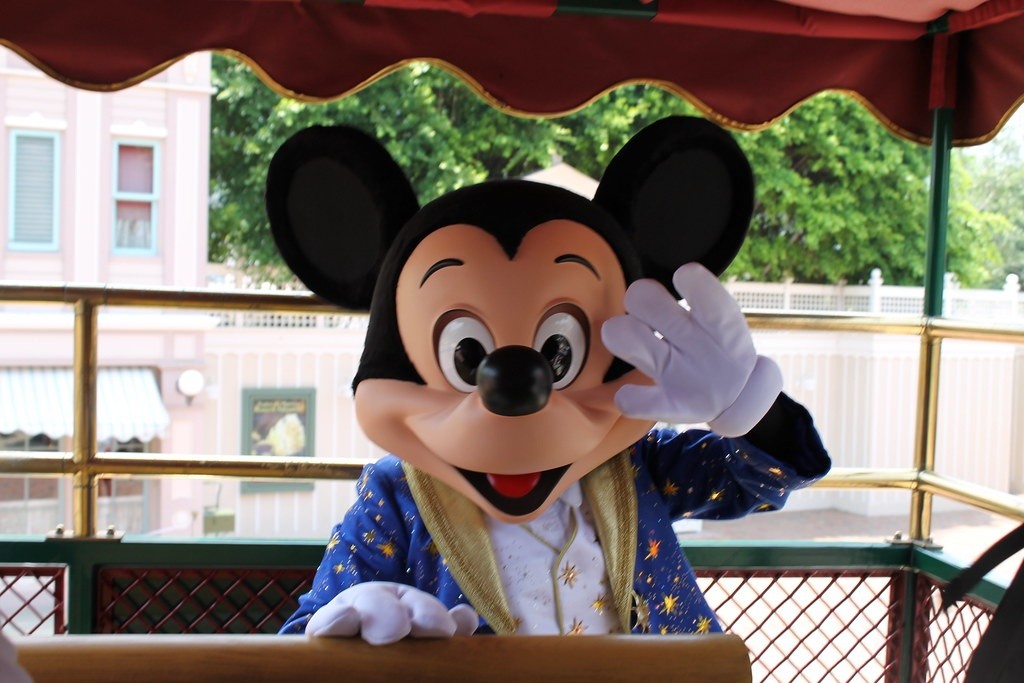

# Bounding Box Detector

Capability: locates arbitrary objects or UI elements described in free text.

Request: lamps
[177,369,205,405]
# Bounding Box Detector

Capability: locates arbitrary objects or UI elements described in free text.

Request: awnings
[0,365,169,445]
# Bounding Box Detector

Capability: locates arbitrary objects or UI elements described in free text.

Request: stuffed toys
[263,115,833,648]
